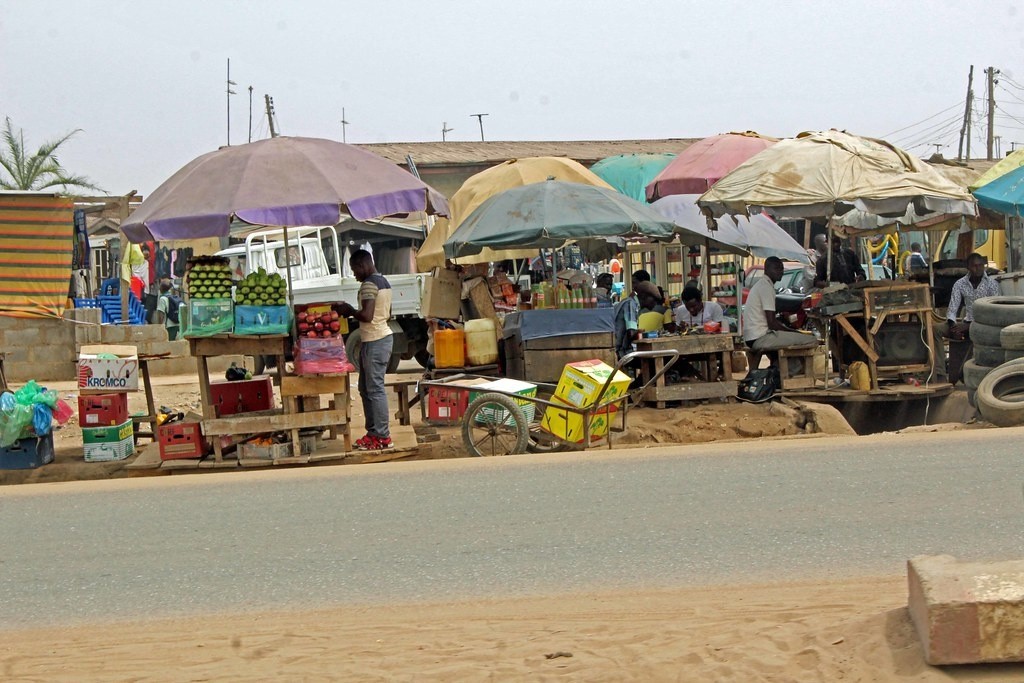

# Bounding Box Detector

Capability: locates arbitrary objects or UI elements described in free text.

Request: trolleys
[419,350,679,457]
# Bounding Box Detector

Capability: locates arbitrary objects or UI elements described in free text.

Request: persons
[157,278,180,340]
[905,242,928,271]
[813,232,866,288]
[741,257,818,375]
[811,234,828,262]
[336,249,394,449]
[637,286,673,335]
[674,286,733,379]
[631,269,650,291]
[615,280,660,387]
[946,252,1002,334]
[597,273,614,294]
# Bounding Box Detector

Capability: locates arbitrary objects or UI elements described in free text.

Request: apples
[297,310,340,338]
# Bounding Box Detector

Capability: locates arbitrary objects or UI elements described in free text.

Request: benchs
[431,365,501,380]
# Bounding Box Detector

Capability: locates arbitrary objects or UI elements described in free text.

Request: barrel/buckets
[849,361,870,390]
[464,318,498,365]
[433,324,464,369]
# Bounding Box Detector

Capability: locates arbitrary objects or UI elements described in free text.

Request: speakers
[870,322,928,366]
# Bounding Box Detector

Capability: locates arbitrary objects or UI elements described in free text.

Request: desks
[287,372,353,456]
[633,334,739,406]
[184,334,293,463]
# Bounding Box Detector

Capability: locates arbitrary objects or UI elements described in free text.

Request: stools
[384,380,419,426]
[742,347,817,390]
[127,361,159,446]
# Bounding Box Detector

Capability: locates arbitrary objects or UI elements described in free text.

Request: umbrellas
[118,136,450,334]
[414,155,671,303]
[595,129,1024,390]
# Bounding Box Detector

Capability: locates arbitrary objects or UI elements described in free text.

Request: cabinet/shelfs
[819,284,933,387]
[504,310,616,381]
[626,239,744,301]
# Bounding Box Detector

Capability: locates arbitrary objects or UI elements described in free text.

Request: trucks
[213,225,435,373]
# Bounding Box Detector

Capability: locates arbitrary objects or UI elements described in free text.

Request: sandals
[359,436,394,450]
[353,435,375,447]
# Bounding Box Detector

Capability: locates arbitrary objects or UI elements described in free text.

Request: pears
[244,370,252,380]
[189,265,232,298]
[235,267,286,305]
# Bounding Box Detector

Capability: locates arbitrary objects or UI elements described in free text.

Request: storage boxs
[78,393,127,427]
[296,339,344,361]
[0,430,54,470]
[237,436,316,459]
[469,378,537,429]
[540,395,616,444]
[429,378,491,419]
[78,345,140,395]
[554,360,633,414]
[210,375,275,418]
[420,276,463,318]
[158,411,204,460]
[236,306,288,334]
[190,299,234,334]
[308,301,350,334]
[82,419,134,463]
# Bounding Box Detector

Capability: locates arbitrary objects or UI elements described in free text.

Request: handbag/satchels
[738,366,777,399]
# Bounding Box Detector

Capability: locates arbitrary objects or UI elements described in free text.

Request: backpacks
[159,294,186,323]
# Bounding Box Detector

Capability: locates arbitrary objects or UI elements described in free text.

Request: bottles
[531,279,597,309]
[904,375,919,386]
